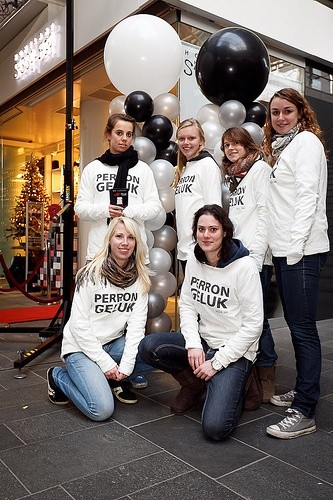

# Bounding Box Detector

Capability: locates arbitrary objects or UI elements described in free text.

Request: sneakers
[129,375,148,388]
[108,378,137,403]
[259,366,276,403]
[46,366,70,404]
[266,407,316,439]
[269,388,297,407]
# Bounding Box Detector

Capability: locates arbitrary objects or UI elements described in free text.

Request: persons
[138,205,264,442]
[220,126,278,400]
[46,218,156,421]
[73,113,162,266]
[262,88,331,440]
[170,117,224,278]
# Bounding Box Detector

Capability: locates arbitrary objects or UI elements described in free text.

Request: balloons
[109,96,127,116]
[153,93,180,120]
[145,205,177,337]
[104,14,186,100]
[149,160,176,190]
[134,123,143,138]
[158,188,175,213]
[195,28,270,107]
[131,136,156,165]
[142,114,173,149]
[196,100,268,165]
[124,91,154,122]
[157,141,179,167]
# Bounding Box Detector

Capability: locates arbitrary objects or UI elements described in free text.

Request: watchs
[211,357,223,371]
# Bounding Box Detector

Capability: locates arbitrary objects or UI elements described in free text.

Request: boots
[170,367,208,416]
[242,365,263,410]
